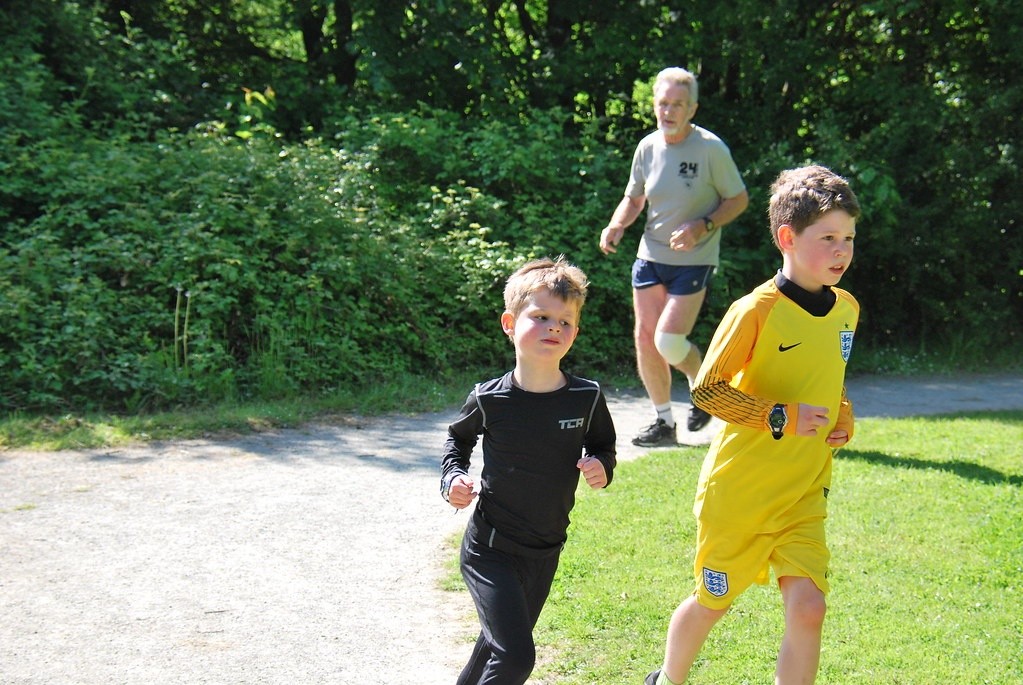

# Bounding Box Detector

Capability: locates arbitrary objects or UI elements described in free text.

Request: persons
[440,252,616,685]
[642,166,860,685]
[599,67,749,446]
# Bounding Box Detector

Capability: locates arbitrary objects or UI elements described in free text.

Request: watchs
[442,472,461,503]
[703,217,714,232]
[770,403,788,440]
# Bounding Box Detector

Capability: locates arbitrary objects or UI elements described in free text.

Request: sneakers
[632,419,678,449]
[687,392,713,432]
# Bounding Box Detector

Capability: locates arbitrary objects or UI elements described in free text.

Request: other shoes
[643,669,663,685]
